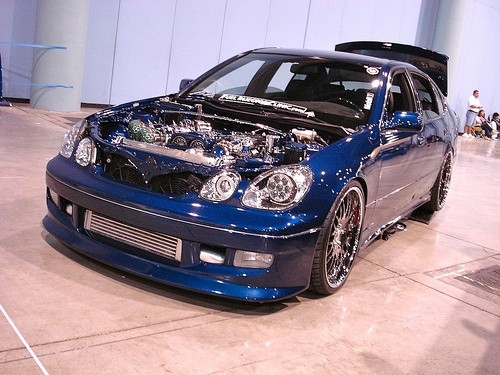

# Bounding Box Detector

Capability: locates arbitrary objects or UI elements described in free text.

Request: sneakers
[462,133,475,139]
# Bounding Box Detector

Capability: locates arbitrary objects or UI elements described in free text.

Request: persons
[491,112,500,137]
[462,90,483,139]
[476,110,500,141]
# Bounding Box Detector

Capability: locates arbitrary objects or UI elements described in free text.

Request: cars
[42,40,458,303]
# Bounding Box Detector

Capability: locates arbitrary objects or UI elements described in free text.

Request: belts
[468,110,477,114]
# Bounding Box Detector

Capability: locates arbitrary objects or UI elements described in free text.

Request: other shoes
[491,131,499,135]
[484,137,491,141]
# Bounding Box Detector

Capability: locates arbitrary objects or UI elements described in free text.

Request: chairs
[355,88,393,121]
[470,124,485,139]
[285,79,345,105]
